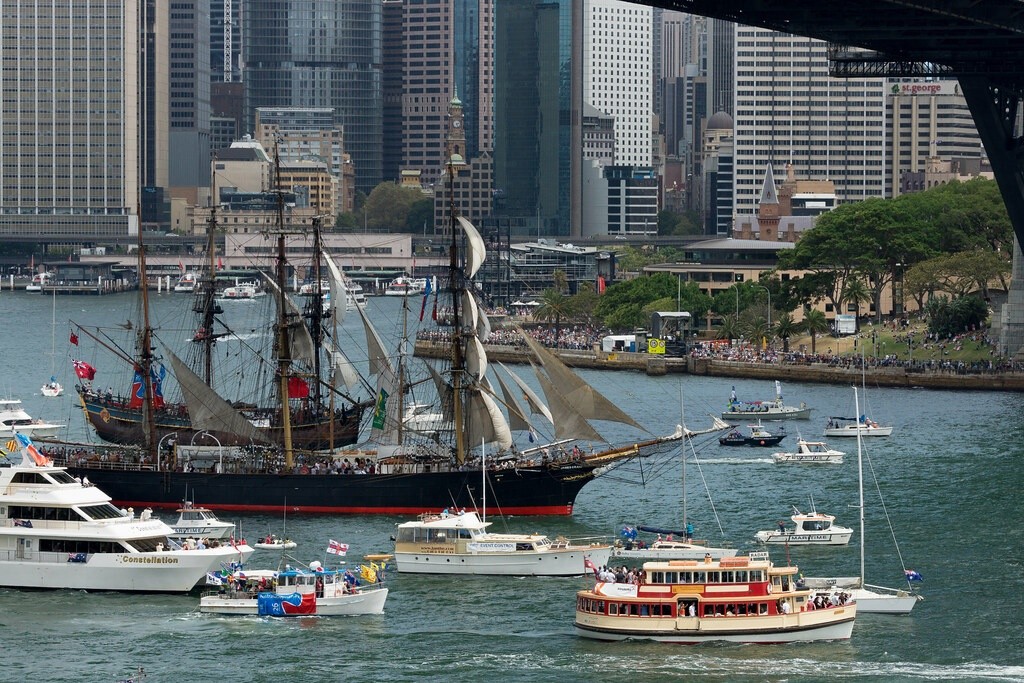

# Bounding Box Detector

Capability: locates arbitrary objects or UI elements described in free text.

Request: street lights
[725,283,738,336]
[751,284,770,331]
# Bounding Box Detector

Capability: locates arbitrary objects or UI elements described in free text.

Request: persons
[814,521,821,530]
[75,382,112,404]
[67,552,75,562]
[680,571,755,584]
[39,445,583,488]
[120,505,153,519]
[258,569,356,598]
[177,533,290,549]
[622,522,693,550]
[677,591,848,619]
[294,404,340,418]
[779,521,785,535]
[419,306,1024,375]
[444,507,466,515]
[597,565,646,584]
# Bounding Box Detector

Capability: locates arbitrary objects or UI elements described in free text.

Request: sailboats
[0,134,744,516]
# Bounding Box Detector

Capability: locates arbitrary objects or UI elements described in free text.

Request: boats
[1,415,255,596]
[395,436,610,574]
[201,498,387,617]
[571,381,857,646]
[719,343,925,615]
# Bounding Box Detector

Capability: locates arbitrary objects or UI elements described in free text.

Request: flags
[69,333,79,345]
[904,569,923,581]
[327,540,349,556]
[586,558,599,580]
[71,359,96,380]
[0,432,46,466]
[361,562,379,584]
[129,363,166,409]
